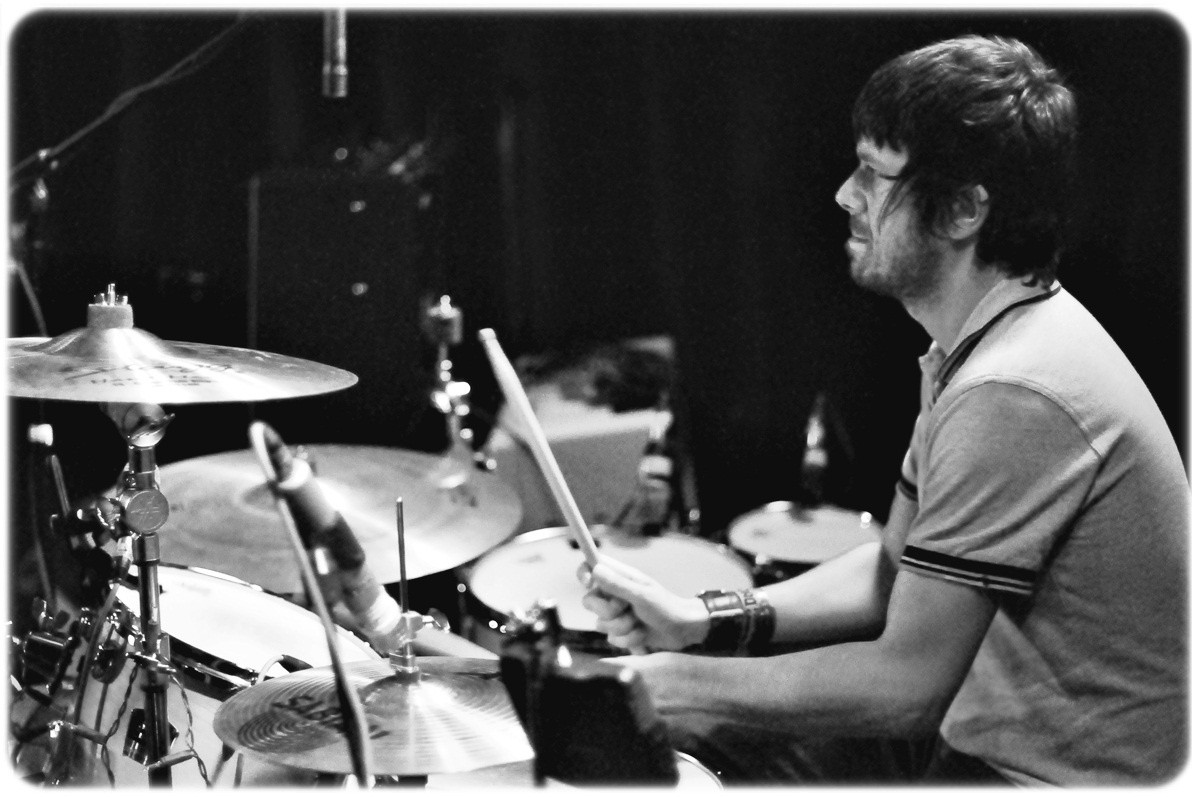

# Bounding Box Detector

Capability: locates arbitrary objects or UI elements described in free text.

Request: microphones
[801,392,829,471]
[262,431,408,657]
[323,7,348,98]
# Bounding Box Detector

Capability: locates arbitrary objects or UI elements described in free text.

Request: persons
[617,375,700,536]
[571,31,1186,787]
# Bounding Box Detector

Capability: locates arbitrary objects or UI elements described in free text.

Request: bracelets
[699,587,777,655]
[684,508,699,526]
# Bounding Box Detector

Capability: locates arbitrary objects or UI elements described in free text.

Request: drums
[462,523,756,675]
[62,560,391,787]
[728,500,884,586]
[336,745,724,788]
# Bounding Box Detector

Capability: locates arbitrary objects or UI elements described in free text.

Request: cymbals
[9,335,359,404]
[213,654,536,776]
[105,444,523,594]
